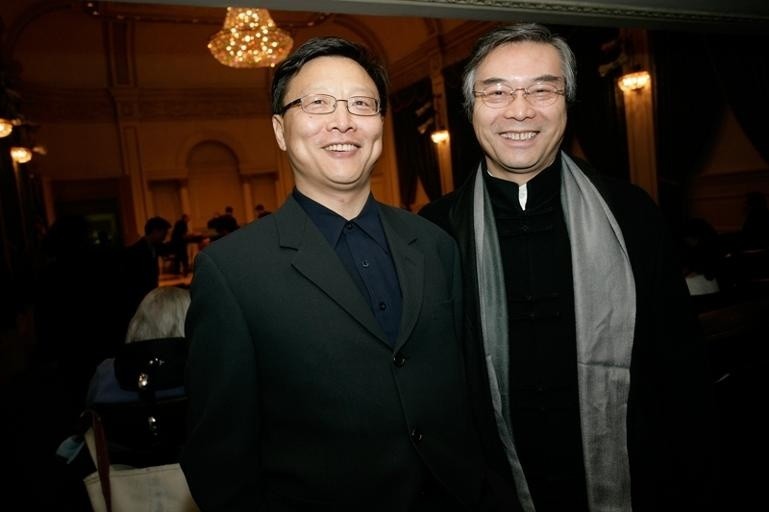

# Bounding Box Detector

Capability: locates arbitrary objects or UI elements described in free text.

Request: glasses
[472,83,564,108]
[279,93,382,116]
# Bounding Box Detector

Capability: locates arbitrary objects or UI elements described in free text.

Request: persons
[30,199,271,288]
[87,286,192,470]
[416,22,708,512]
[187,38,506,512]
